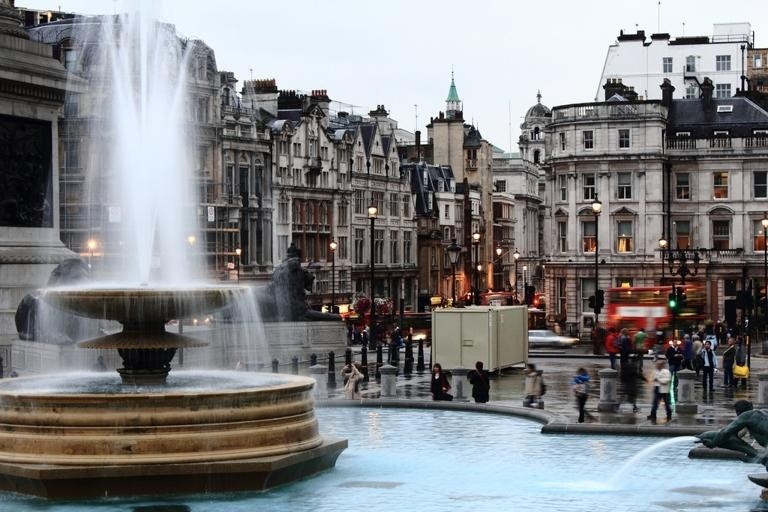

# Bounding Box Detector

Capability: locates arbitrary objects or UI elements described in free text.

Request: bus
[604,285,705,354]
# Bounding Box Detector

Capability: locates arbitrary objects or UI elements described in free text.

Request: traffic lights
[588,296,596,309]
[677,287,687,308]
[595,289,604,312]
[668,293,676,313]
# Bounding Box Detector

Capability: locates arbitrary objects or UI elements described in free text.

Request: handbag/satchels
[733,363,749,378]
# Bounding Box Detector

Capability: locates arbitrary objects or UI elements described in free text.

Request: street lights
[762,211,768,355]
[330,236,339,314]
[235,242,241,283]
[443,229,545,311]
[87,239,97,269]
[659,233,668,283]
[590,192,602,329]
[662,246,703,288]
[367,189,379,350]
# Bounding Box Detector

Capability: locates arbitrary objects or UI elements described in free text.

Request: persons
[286,242,300,259]
[703,400,768,472]
[386,326,400,364]
[573,368,589,423]
[524,363,543,408]
[430,363,453,401]
[470,361,490,403]
[590,318,746,420]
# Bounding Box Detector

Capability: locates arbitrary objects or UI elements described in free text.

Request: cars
[527,329,578,349]
